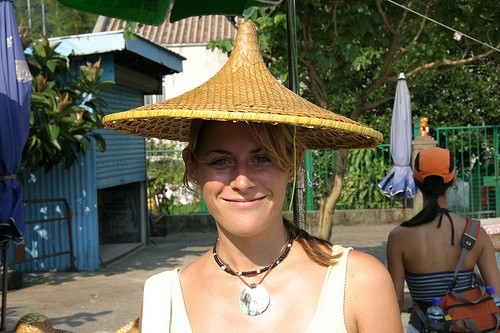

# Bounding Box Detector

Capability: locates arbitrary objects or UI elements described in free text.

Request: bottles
[426,297,444,321]
[486,286,500,309]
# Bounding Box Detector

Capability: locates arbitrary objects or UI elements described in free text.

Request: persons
[386,147,500,333]
[102,22,404,333]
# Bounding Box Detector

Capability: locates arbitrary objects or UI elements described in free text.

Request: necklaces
[213,225,294,316]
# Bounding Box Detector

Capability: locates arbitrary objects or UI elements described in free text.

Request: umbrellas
[377,73,417,224]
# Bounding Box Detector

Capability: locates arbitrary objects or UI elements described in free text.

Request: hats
[102,18,384,149]
[412,147,456,183]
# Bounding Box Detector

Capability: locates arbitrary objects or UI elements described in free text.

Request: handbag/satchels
[440,287,500,333]
